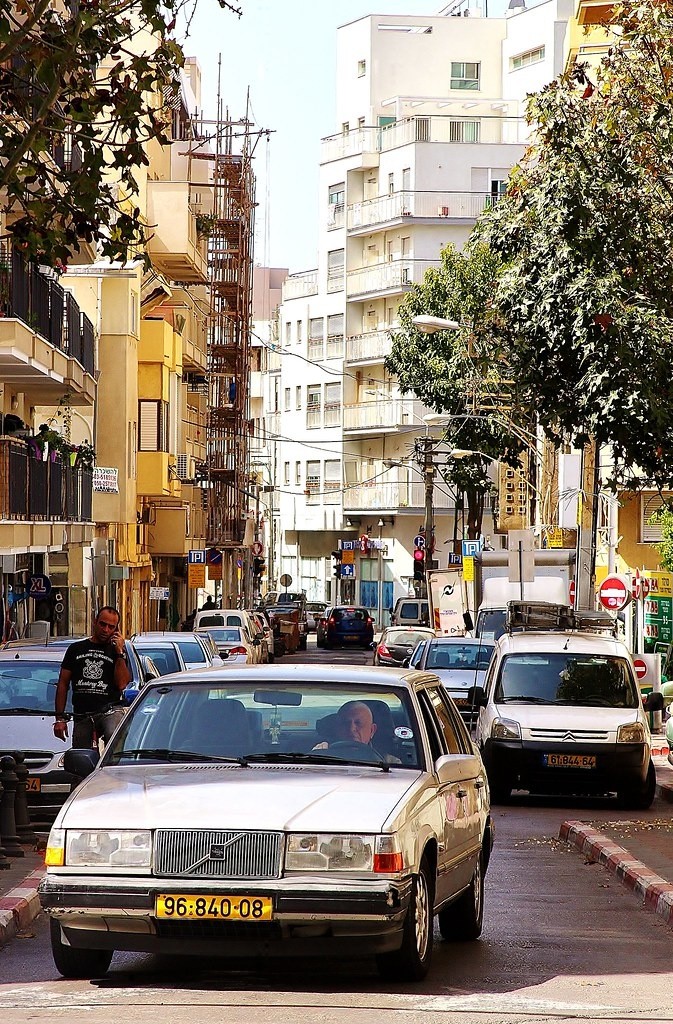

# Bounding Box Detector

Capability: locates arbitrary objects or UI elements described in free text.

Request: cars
[195,626,261,664]
[246,611,277,664]
[0,631,229,675]
[467,631,664,811]
[405,638,497,731]
[38,663,492,981]
[138,653,162,683]
[259,591,328,634]
[314,605,375,650]
[0,647,142,816]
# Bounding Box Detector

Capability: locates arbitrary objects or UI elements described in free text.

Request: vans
[194,610,265,664]
[389,599,429,627]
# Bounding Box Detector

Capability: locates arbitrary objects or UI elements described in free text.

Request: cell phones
[113,629,119,645]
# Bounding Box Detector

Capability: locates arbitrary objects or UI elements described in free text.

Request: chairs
[39,678,73,712]
[390,710,413,754]
[474,650,489,668]
[153,658,167,674]
[245,709,262,754]
[433,652,453,667]
[180,698,251,756]
[226,632,238,641]
[333,700,411,767]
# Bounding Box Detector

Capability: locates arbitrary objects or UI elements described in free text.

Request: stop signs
[568,582,575,605]
[633,659,647,680]
[632,579,650,600]
[597,579,628,609]
[635,567,641,597]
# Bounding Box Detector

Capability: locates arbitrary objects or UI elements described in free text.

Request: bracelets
[56,712,65,719]
[52,720,66,725]
[116,653,126,658]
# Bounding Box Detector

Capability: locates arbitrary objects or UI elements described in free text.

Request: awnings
[141,263,173,321]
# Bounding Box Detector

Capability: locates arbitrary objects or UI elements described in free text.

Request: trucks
[426,551,576,638]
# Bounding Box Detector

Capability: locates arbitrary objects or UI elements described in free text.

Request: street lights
[385,412,557,550]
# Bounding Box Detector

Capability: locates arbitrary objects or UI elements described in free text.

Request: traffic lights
[332,549,341,580]
[253,558,265,575]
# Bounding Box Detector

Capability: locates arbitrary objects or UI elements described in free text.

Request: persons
[54,607,132,792]
[312,700,403,764]
[186,609,196,628]
[202,596,219,610]
